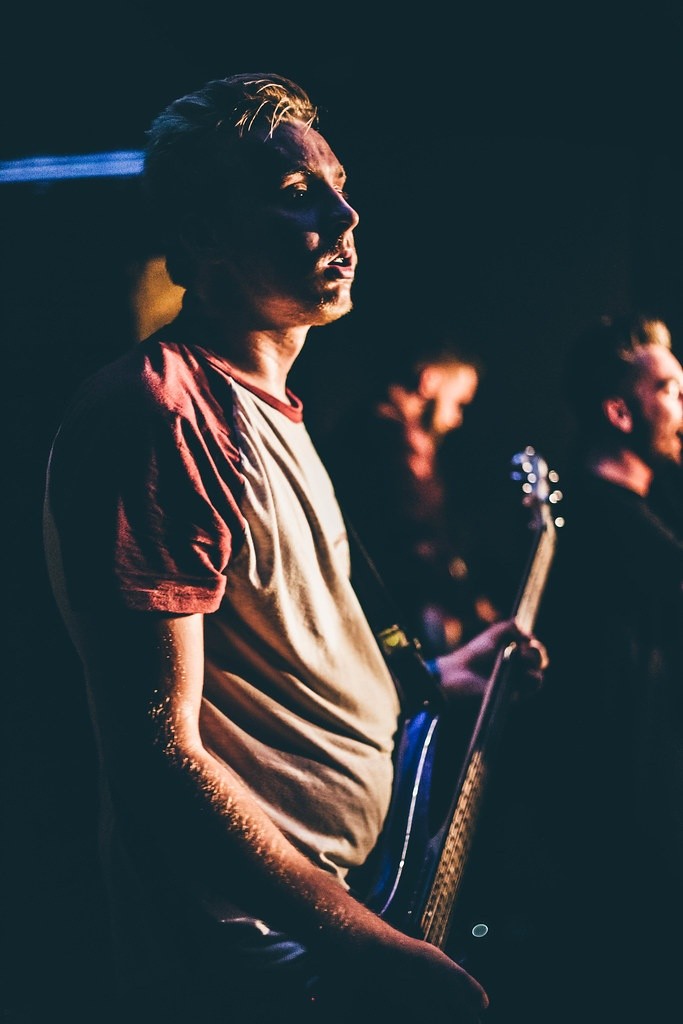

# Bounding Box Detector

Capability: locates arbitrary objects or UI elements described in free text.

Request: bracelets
[426,659,441,684]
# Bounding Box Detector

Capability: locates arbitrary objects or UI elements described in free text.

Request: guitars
[310,438,567,1024]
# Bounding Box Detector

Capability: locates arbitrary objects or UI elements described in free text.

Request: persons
[42,72,548,1024]
[575,314,682,548]
